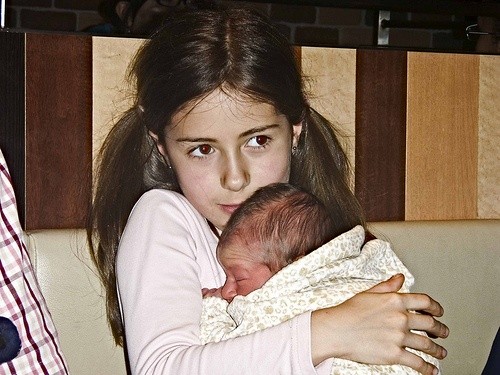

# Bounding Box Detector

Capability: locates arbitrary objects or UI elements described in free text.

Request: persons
[0,140,70,375]
[201,182,443,374]
[80,5,451,375]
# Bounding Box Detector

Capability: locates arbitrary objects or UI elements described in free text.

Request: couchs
[28,220,497,375]
